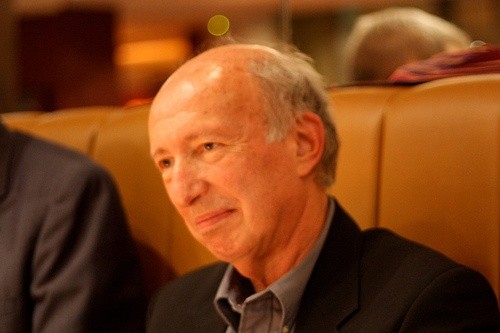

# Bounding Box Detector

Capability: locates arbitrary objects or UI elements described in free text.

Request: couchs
[0,73,500,305]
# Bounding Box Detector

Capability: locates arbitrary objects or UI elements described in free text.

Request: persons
[339,8,471,81]
[140,39,500,333]
[0,122,147,333]
